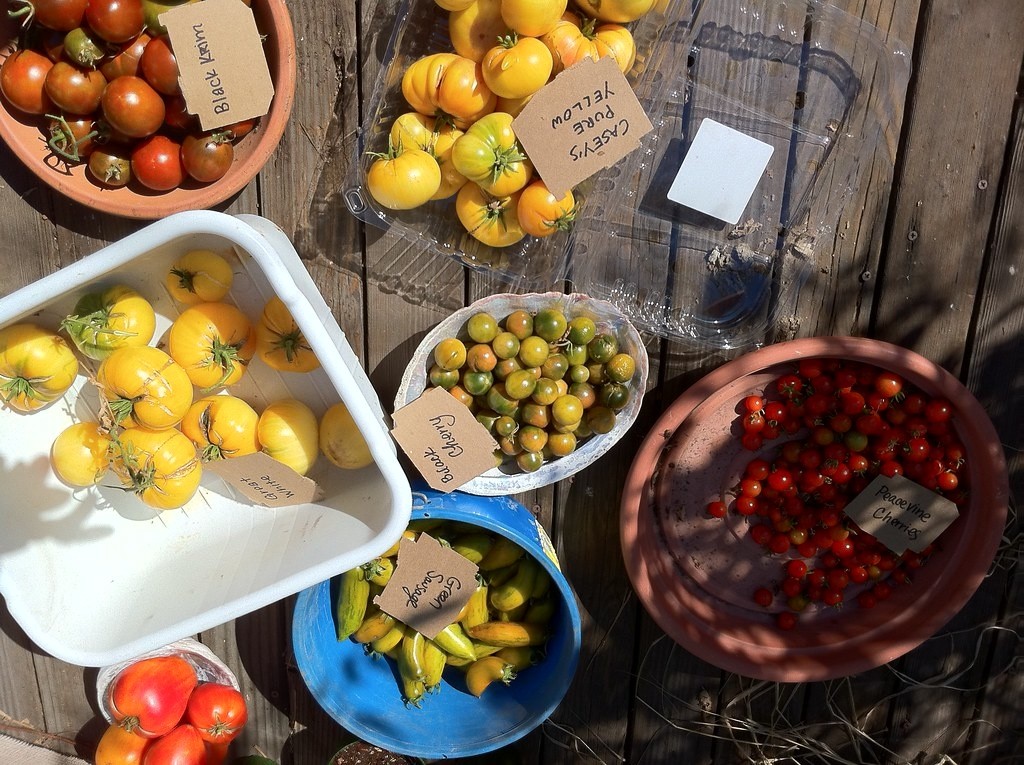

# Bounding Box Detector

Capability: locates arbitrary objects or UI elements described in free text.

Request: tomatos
[4,0,966,764]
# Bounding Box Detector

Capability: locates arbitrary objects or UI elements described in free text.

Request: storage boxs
[0,209,414,670]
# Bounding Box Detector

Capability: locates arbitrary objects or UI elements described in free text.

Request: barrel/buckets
[287,491,583,762]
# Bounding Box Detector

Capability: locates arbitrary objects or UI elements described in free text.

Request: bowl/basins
[618,333,1009,684]
[0,210,413,669]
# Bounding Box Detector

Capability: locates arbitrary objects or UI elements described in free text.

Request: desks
[1,0,1019,760]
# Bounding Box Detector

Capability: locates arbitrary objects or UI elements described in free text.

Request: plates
[96,637,241,743]
[346,1,912,356]
[0,1,298,221]
[391,288,652,497]
